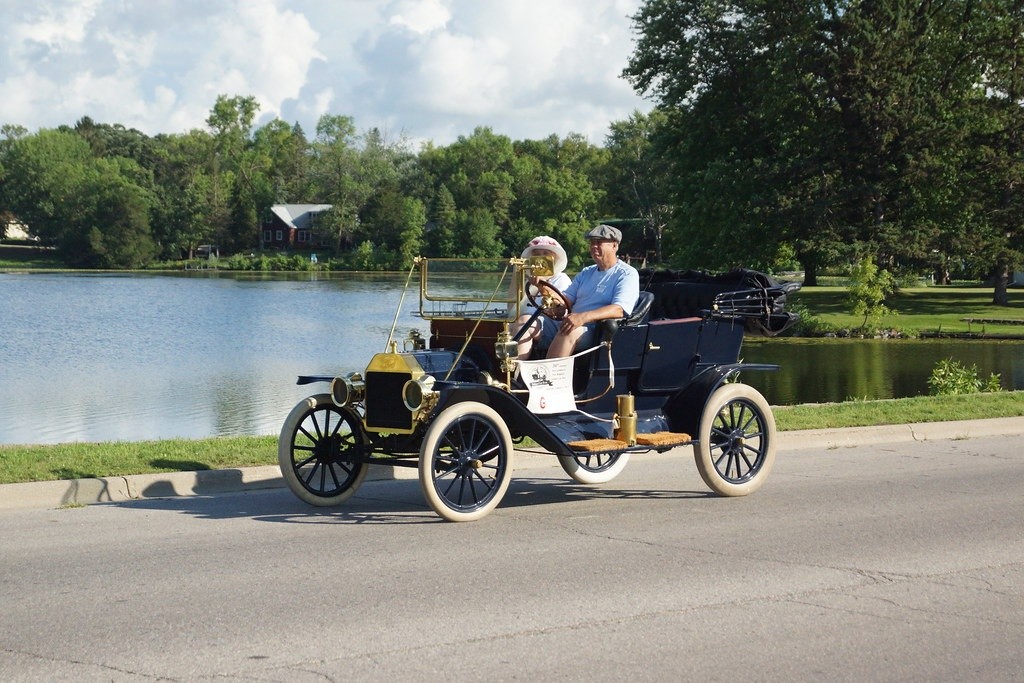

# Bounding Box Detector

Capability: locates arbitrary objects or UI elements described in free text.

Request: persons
[508,236,572,361]
[528,225,640,358]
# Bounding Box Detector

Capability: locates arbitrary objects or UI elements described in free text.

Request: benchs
[960,318,1024,336]
[635,280,757,325]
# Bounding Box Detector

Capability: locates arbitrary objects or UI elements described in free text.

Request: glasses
[589,240,614,243]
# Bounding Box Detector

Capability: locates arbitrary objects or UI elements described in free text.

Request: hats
[588,225,622,243]
[521,236,567,275]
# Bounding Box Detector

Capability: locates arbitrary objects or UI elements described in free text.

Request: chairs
[589,290,654,370]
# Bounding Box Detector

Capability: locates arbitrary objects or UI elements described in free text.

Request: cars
[278,254,802,523]
